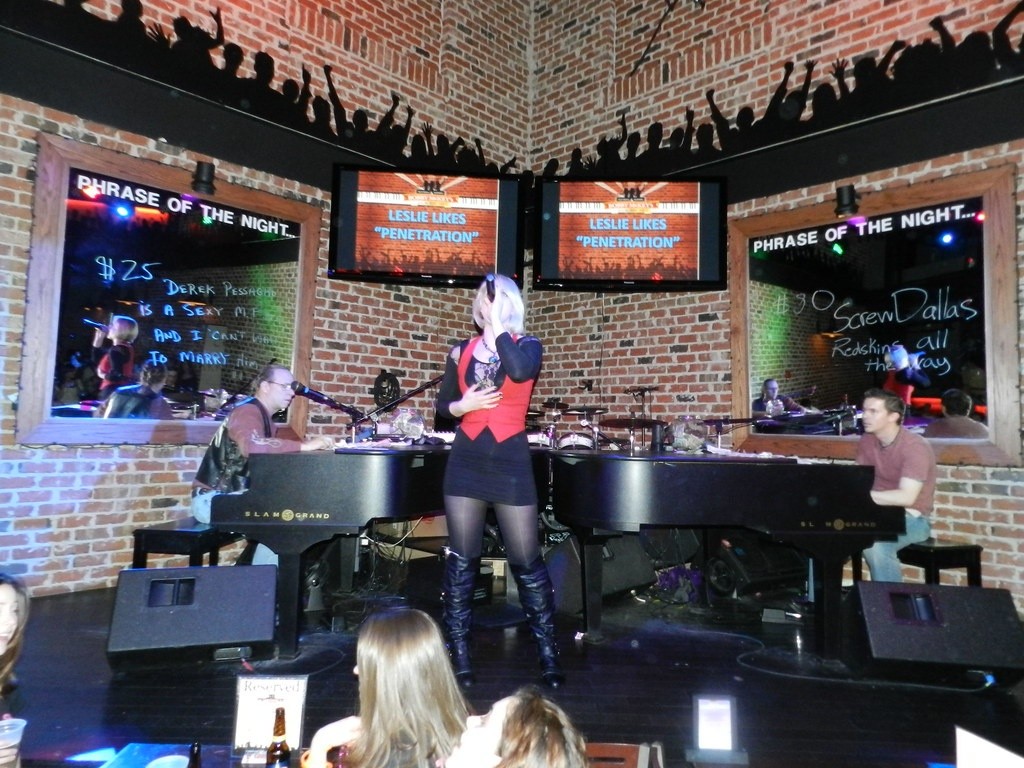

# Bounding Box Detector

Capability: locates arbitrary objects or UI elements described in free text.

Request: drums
[526,429,556,447]
[595,439,631,450]
[557,433,594,449]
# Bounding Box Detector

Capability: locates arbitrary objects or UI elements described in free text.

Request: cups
[0,719,27,768]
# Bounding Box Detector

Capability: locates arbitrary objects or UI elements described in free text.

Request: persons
[190,365,332,566]
[166,366,177,389]
[883,345,915,417]
[304,608,469,768]
[857,388,936,582]
[751,379,806,421]
[924,390,990,439]
[444,686,588,768]
[0,572,30,768]
[60,364,85,407]
[92,360,175,420]
[91,315,138,402]
[436,274,564,690]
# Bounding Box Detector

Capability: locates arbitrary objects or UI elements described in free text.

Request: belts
[191,488,210,498]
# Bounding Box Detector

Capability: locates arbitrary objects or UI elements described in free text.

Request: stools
[852,538,983,587]
[132,515,248,568]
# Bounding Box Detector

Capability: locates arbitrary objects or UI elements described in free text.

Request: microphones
[485,273,495,303]
[83,318,108,333]
[290,381,333,403]
[915,351,926,357]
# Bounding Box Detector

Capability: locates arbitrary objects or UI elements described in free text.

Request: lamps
[834,185,861,218]
[191,161,217,196]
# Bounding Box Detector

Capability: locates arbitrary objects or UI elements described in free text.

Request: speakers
[717,538,810,594]
[542,532,657,614]
[106,564,276,674]
[835,579,1024,690]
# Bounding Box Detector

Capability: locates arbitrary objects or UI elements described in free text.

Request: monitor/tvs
[532,175,728,293]
[327,163,527,289]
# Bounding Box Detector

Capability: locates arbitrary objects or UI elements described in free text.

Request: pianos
[754,410,864,435]
[552,449,907,652]
[208,446,549,662]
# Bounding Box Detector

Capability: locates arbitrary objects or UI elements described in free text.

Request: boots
[508,553,566,689]
[441,544,482,690]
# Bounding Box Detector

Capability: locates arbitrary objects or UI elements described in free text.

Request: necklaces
[482,339,499,363]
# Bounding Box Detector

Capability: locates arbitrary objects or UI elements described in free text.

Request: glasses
[268,381,292,391]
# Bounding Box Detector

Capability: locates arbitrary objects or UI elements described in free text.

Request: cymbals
[527,410,546,417]
[562,408,609,414]
[598,418,668,428]
[541,402,568,409]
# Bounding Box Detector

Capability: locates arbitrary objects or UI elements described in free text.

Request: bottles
[390,409,424,441]
[204,389,229,411]
[670,416,707,451]
[188,742,201,768]
[267,708,290,768]
